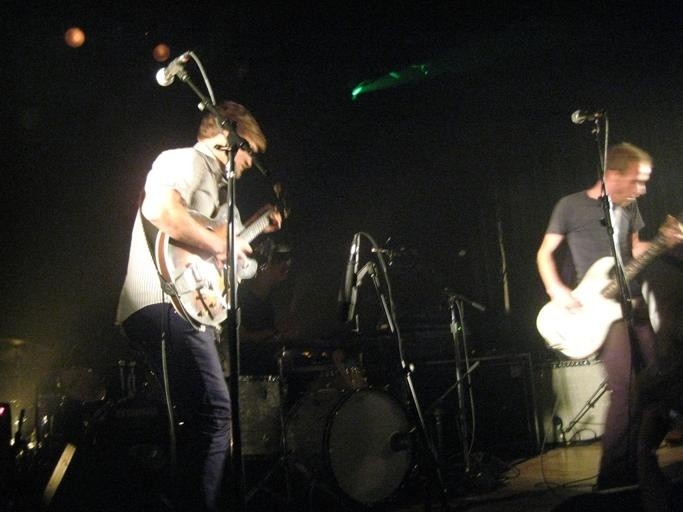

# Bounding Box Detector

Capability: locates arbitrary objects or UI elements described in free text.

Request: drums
[295,360,367,397]
[280,376,415,512]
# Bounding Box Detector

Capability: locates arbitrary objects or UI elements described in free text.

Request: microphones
[336,233,361,323]
[155,51,189,86]
[570,109,603,124]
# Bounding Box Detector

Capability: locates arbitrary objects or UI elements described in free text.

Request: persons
[114,102,282,512]
[537,142,683,488]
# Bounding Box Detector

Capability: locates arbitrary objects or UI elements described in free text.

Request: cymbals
[249,239,292,257]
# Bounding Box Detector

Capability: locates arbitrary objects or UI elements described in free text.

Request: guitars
[537,213,683,360]
[153,190,293,330]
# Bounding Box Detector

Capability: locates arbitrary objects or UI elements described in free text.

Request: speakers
[413,351,541,460]
[531,359,609,444]
